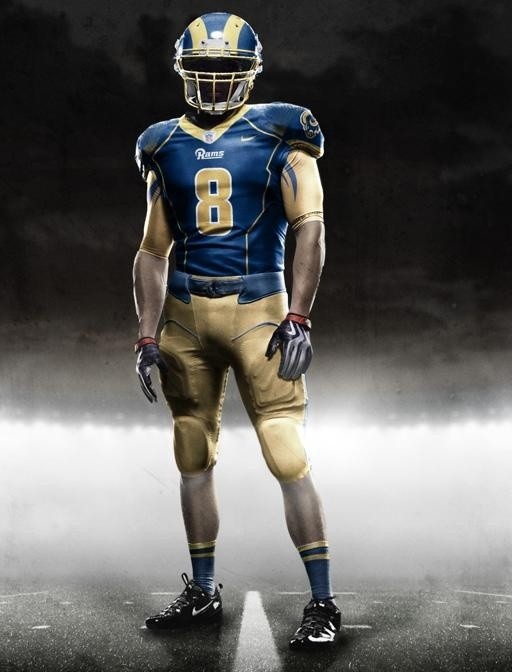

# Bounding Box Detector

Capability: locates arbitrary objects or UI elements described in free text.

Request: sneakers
[145,572,224,632]
[289,598,342,652]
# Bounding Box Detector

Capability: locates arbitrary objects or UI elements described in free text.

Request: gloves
[265,313,314,381]
[134,336,166,404]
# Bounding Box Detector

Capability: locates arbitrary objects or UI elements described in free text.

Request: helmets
[173,11,264,74]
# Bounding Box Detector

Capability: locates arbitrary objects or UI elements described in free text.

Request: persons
[132,12,342,652]
